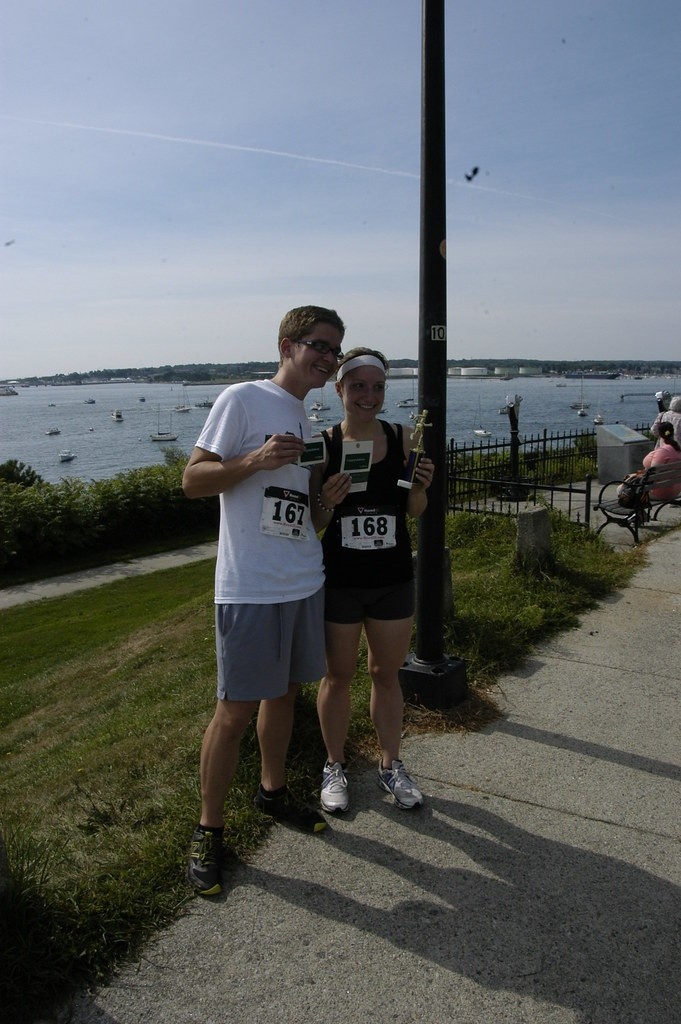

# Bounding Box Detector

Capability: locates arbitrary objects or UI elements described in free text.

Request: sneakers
[187,830,222,895]
[253,783,327,832]
[377,757,424,811]
[320,758,350,813]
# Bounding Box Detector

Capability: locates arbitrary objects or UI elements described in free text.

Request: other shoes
[624,514,648,528]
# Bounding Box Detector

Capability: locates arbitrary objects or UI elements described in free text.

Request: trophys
[397,410,433,489]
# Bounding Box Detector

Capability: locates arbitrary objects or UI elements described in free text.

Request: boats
[0,372,646,463]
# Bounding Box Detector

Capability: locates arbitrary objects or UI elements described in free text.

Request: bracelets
[316,491,335,511]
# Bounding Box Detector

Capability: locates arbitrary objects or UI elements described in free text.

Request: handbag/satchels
[618,473,652,523]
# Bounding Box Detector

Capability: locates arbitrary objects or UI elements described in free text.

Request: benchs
[593,461,681,544]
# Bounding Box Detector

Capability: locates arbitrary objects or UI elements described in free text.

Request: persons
[309,347,435,812]
[182,306,345,896]
[627,396,681,528]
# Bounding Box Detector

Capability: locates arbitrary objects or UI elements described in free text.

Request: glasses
[339,382,388,393]
[293,339,345,362]
